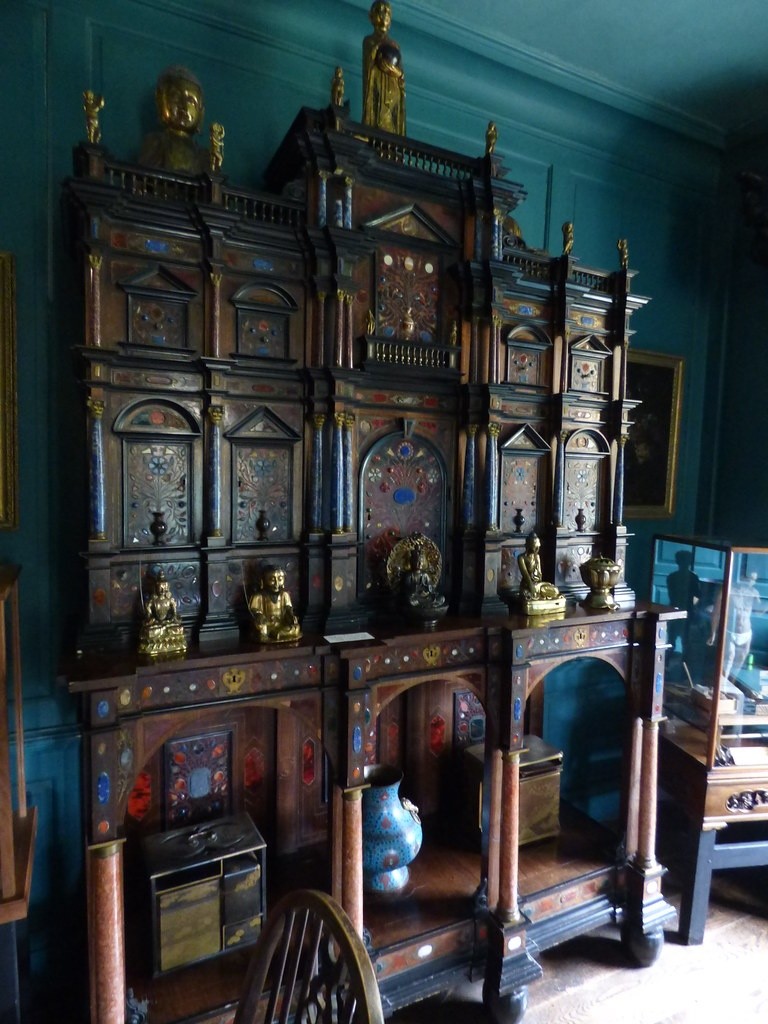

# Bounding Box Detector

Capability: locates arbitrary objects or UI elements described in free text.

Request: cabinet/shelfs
[649,533,768,947]
[51,103,688,1024]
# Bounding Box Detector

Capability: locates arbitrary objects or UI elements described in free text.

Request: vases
[362,762,423,892]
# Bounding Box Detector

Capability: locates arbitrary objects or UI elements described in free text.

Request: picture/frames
[623,347,686,519]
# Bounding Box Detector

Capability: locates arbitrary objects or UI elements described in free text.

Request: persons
[625,436,666,504]
[248,566,299,637]
[82,88,104,143]
[143,580,185,636]
[209,120,225,172]
[399,550,446,607]
[517,533,560,600]
[331,65,345,106]
[617,238,629,269]
[486,121,498,152]
[362,0,406,136]
[664,554,700,666]
[136,65,209,179]
[562,221,574,254]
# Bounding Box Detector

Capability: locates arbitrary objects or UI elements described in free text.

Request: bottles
[363,764,422,893]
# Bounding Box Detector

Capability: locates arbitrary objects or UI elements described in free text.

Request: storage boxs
[464,733,563,848]
